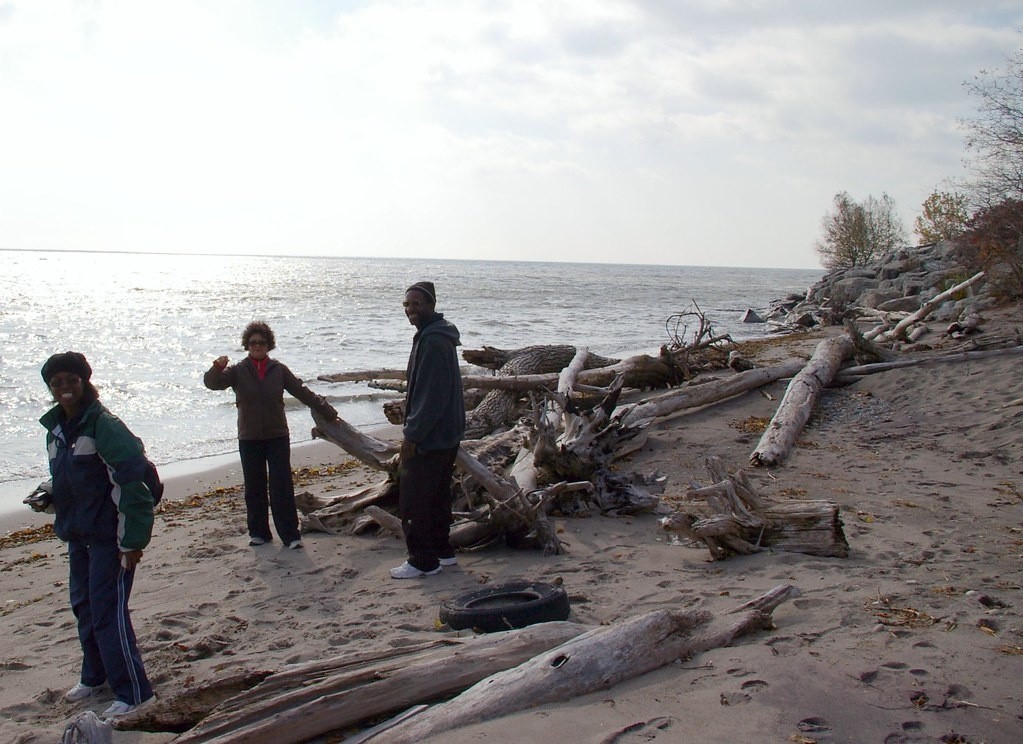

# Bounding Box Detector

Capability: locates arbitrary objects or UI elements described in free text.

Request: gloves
[318,401,339,421]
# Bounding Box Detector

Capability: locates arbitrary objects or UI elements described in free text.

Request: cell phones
[28,491,47,500]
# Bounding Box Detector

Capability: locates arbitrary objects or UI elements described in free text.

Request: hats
[42,352,92,386]
[406,281,436,305]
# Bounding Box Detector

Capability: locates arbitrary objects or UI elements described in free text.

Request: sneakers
[289,540,303,548]
[438,557,457,566]
[65,681,109,702]
[103,695,156,719]
[390,560,443,578]
[249,537,264,546]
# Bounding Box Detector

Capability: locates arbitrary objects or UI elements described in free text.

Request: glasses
[403,300,430,307]
[247,340,269,347]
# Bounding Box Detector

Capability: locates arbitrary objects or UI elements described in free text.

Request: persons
[23,351,160,719]
[389,281,470,579]
[204,321,340,552]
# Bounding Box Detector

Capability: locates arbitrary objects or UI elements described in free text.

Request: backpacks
[92,406,164,507]
